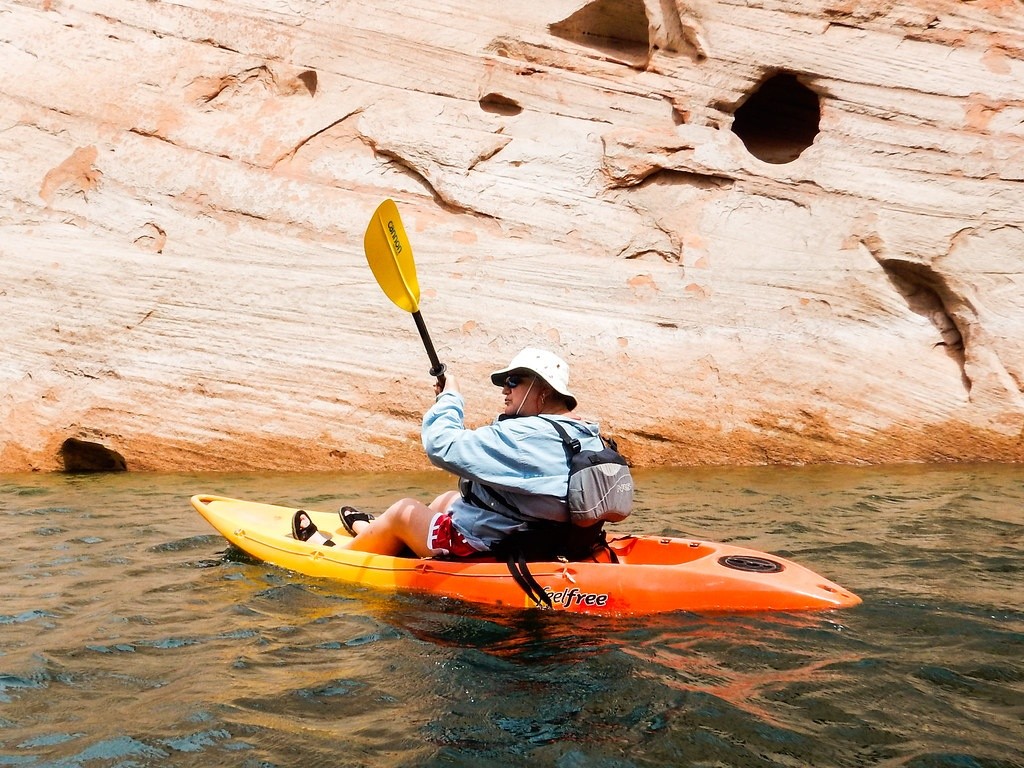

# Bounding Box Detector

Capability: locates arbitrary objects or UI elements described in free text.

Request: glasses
[505,374,531,388]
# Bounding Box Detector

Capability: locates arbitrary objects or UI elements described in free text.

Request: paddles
[362,197,445,388]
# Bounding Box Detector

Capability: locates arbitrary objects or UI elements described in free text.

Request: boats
[190,492,865,614]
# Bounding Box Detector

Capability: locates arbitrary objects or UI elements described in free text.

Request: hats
[491,347,579,412]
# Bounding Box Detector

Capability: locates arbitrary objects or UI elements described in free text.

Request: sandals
[292,510,337,547]
[339,506,375,538]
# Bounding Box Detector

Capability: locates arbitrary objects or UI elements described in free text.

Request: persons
[291,346,608,563]
